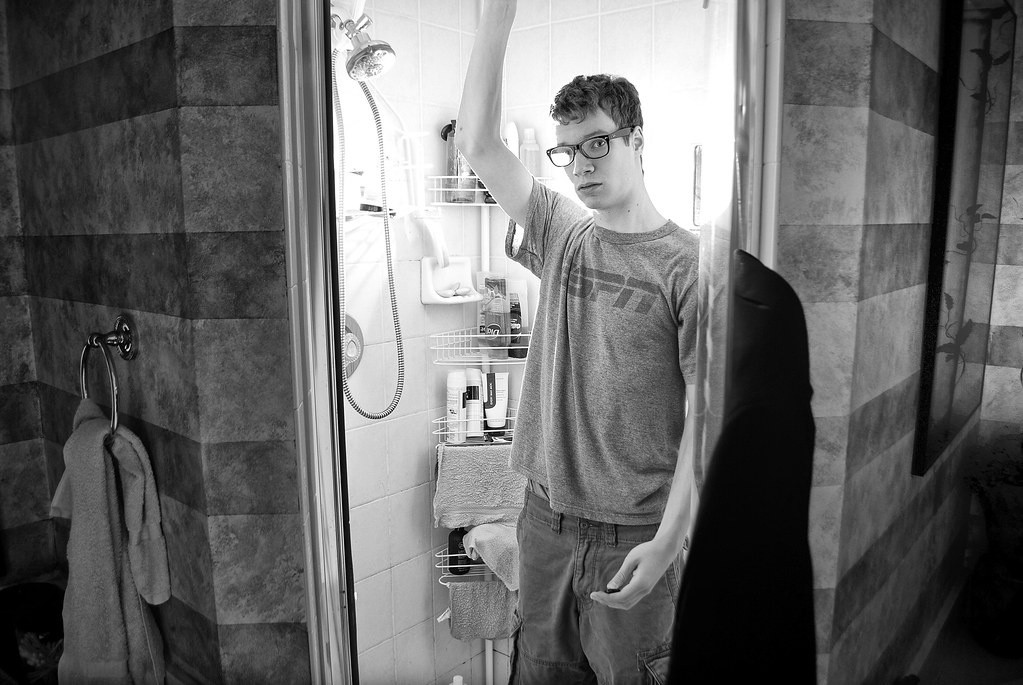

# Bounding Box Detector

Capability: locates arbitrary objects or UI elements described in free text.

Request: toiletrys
[445,370,466,445]
[441,118,476,203]
[519,128,542,174]
[502,120,519,165]
[476,269,497,355]
[506,291,528,358]
[461,367,485,438]
[484,276,509,359]
[480,371,510,435]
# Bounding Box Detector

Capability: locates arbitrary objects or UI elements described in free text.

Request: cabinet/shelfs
[420,171,549,587]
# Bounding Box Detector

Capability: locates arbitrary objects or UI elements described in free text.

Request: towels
[434,442,531,530]
[462,519,532,590]
[48,394,175,685]
[438,580,521,642]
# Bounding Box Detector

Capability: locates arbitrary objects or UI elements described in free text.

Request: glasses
[547,126,635,167]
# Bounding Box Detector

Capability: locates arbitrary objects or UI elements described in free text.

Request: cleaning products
[447,524,472,575]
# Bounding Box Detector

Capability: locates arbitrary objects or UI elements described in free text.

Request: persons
[455,1,733,685]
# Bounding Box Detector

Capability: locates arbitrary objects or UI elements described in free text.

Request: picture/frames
[910,0,1020,477]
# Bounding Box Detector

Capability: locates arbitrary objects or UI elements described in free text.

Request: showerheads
[332,9,406,421]
